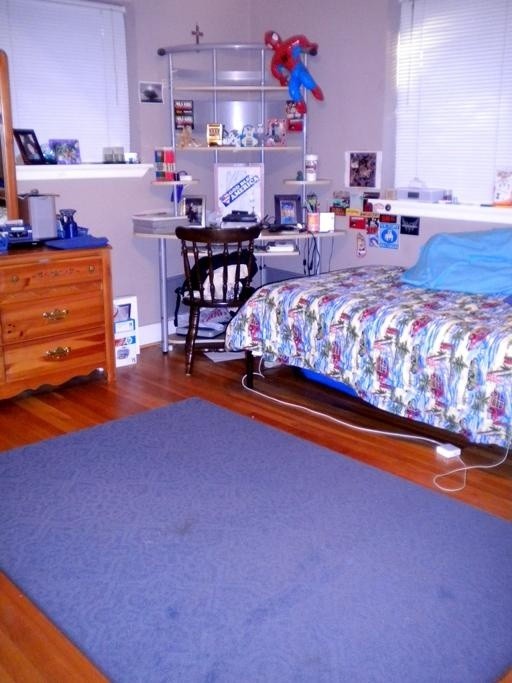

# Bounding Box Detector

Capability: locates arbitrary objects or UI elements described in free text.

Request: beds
[225,265,512,451]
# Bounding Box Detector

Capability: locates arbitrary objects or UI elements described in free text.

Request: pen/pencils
[303,198,319,214]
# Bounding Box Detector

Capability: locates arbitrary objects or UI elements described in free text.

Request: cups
[304,154,317,180]
[307,213,320,232]
[103,147,125,163]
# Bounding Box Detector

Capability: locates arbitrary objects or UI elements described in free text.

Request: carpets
[0,397,512,683]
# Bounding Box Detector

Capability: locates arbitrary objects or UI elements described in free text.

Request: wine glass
[63,211,79,239]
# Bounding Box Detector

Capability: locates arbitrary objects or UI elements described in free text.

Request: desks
[134,43,346,353]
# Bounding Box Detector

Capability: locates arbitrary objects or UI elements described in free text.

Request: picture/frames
[345,150,381,190]
[175,100,193,130]
[214,163,263,224]
[182,196,206,229]
[113,296,140,354]
[15,129,47,164]
[274,194,302,226]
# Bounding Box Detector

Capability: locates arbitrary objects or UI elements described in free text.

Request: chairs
[175,226,261,377]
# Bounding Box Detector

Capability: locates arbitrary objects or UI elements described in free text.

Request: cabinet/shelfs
[0,245,117,401]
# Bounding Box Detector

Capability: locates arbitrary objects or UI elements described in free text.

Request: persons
[263,29,323,115]
[22,139,39,159]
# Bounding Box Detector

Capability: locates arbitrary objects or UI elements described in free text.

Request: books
[131,211,190,234]
[265,241,294,253]
